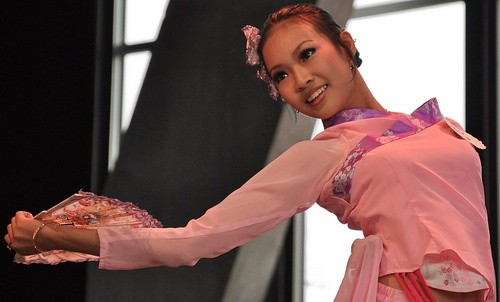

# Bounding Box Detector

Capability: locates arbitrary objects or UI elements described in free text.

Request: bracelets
[33,221,60,253]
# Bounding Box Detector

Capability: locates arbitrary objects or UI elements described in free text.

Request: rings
[6,243,11,249]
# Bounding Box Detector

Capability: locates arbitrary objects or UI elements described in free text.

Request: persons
[3,4,498,302]
